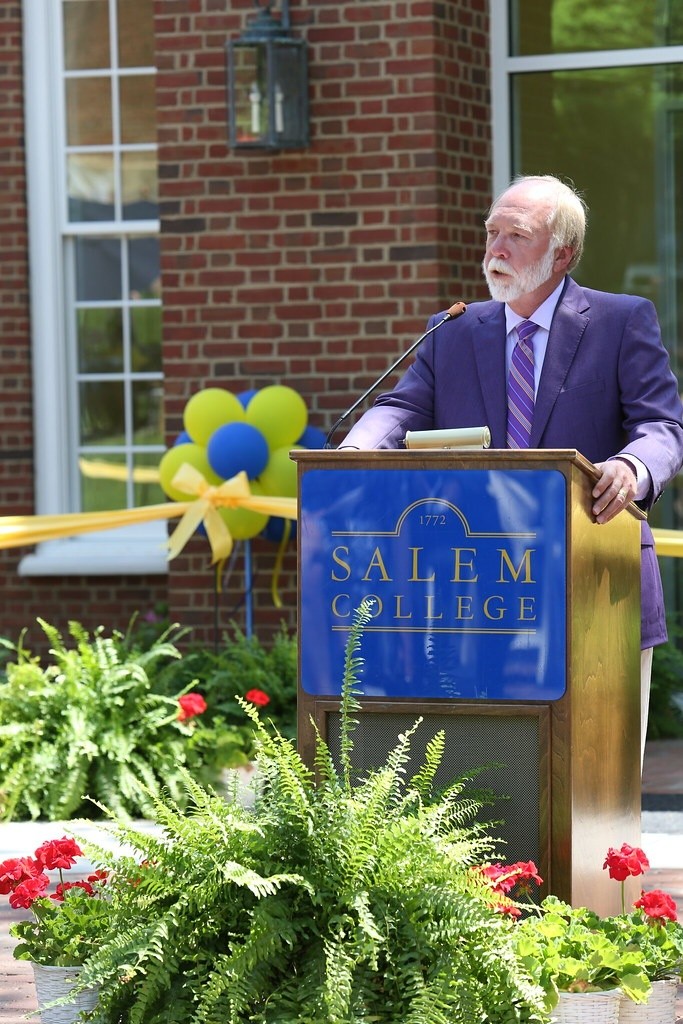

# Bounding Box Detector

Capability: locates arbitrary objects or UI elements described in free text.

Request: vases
[619,975,678,1024]
[31,962,100,1024]
[548,988,624,1024]
[220,762,263,810]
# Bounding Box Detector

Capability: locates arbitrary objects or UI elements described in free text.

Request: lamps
[225,0,310,153]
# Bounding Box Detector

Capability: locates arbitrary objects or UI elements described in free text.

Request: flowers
[178,689,277,793]
[0,836,158,968]
[466,843,683,1017]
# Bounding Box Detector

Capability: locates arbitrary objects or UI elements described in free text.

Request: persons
[339,176,682,774]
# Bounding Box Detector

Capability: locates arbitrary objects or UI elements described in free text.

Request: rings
[619,490,628,497]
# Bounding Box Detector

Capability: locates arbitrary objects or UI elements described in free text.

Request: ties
[505,319,540,449]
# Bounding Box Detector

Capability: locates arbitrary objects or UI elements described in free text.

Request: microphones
[322,301,467,449]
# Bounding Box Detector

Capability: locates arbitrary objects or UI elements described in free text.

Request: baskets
[619,979,677,1024]
[546,987,622,1024]
[31,962,112,1024]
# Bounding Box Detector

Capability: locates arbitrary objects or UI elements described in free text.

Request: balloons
[247,386,307,446]
[171,390,328,539]
[183,388,247,446]
[208,423,268,481]
[158,443,221,504]
